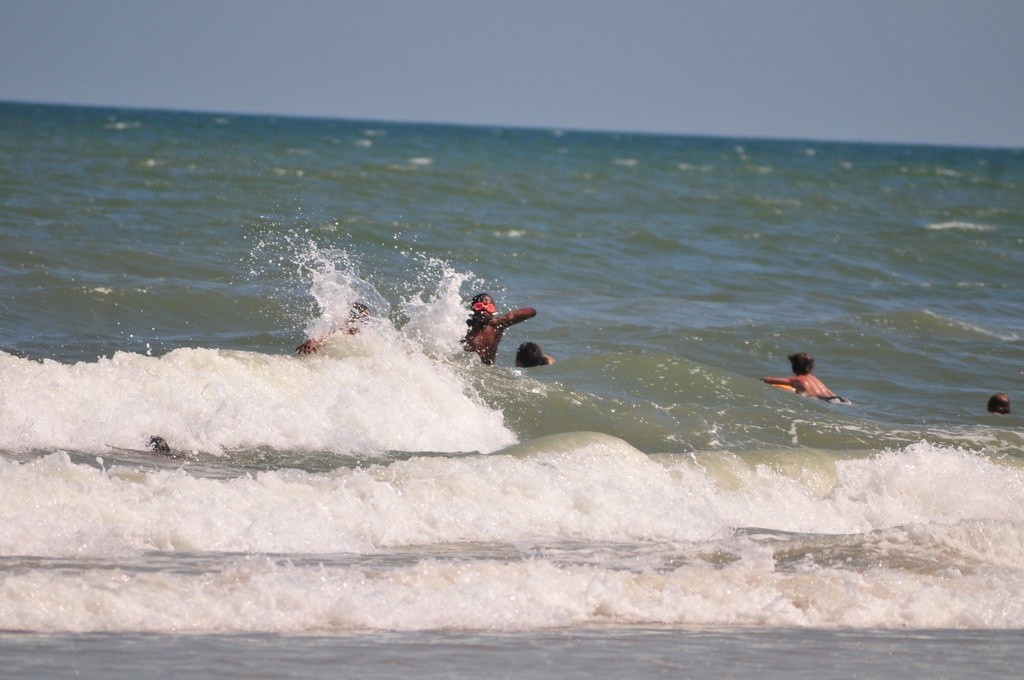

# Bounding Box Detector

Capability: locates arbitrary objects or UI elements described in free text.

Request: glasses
[471,302,495,313]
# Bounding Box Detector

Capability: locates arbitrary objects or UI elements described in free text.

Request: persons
[294,302,371,356]
[761,353,854,405]
[515,341,556,368]
[458,293,537,365]
